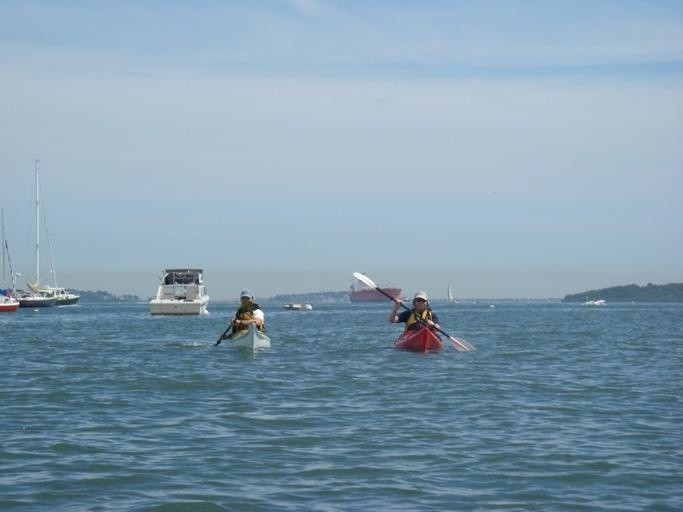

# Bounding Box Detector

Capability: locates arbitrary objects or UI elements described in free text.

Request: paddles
[353,270,476,352]
[214,301,259,346]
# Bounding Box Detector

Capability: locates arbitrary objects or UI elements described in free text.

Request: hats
[415,291,428,301]
[240,288,255,299]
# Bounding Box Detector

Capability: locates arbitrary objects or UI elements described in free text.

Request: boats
[348,288,401,302]
[583,299,606,306]
[216,323,270,349]
[394,327,443,354]
[284,303,311,310]
[148,268,210,315]
[403,296,410,302]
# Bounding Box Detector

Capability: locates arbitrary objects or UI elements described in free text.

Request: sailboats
[0,160,80,311]
[447,284,457,303]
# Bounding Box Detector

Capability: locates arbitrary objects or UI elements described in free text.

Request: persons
[220,289,265,340]
[389,291,440,342]
